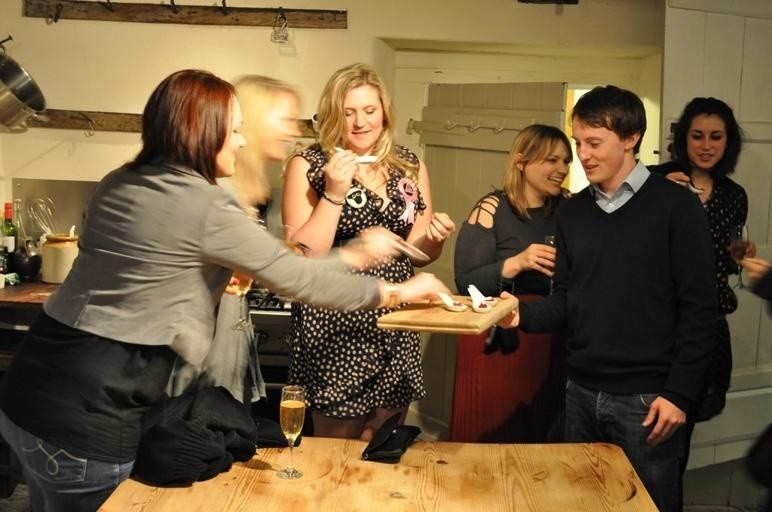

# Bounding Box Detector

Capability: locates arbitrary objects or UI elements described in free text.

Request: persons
[455,125,574,443]
[165,74,402,405]
[739,256,772,512]
[1,68,455,512]
[281,65,455,442]
[644,97,758,315]
[496,86,716,512]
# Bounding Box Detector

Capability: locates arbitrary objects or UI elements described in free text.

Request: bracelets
[322,192,346,205]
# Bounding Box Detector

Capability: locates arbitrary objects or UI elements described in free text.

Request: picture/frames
[93,436,659,511]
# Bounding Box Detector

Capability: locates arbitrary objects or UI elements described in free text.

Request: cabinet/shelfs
[41,234,80,284]
[1,201,17,268]
[545,236,555,297]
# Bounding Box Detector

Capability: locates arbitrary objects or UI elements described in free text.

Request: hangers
[332,146,381,164]
[439,284,497,313]
[688,183,704,196]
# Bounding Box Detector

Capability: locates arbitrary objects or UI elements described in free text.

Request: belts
[729,239,756,290]
[277,383,308,479]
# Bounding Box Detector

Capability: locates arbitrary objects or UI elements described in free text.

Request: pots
[1,44,50,136]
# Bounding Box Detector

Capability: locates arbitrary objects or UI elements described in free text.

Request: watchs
[384,282,399,308]
